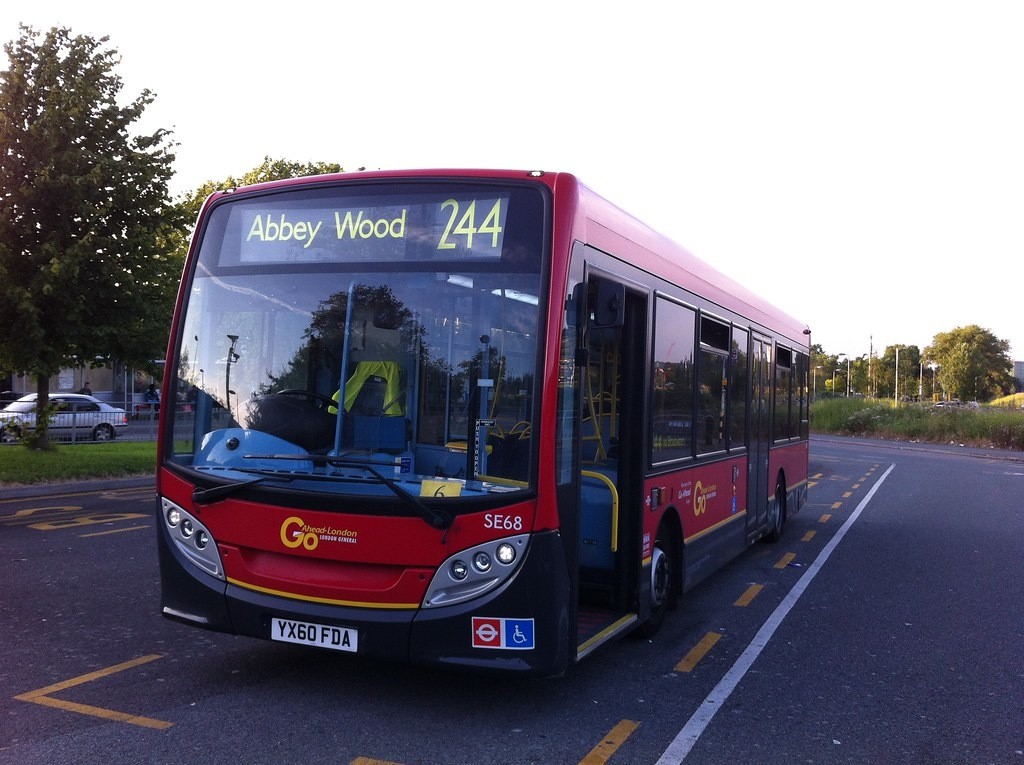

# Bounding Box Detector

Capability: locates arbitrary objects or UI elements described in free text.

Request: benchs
[134,404,192,422]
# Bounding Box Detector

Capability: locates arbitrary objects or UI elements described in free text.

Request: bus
[154,165,813,679]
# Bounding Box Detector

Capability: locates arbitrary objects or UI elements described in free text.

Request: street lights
[228,389,240,425]
[814,366,824,402]
[866,351,878,398]
[832,368,843,398]
[838,352,850,398]
[199,369,206,391]
[191,336,200,385]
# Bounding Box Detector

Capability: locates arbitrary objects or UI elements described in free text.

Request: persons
[135,371,144,389]
[146,385,160,411]
[80,382,93,396]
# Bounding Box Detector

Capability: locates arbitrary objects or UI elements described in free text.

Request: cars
[0,391,130,442]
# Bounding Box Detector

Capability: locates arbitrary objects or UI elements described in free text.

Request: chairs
[329,361,407,448]
[583,374,620,421]
